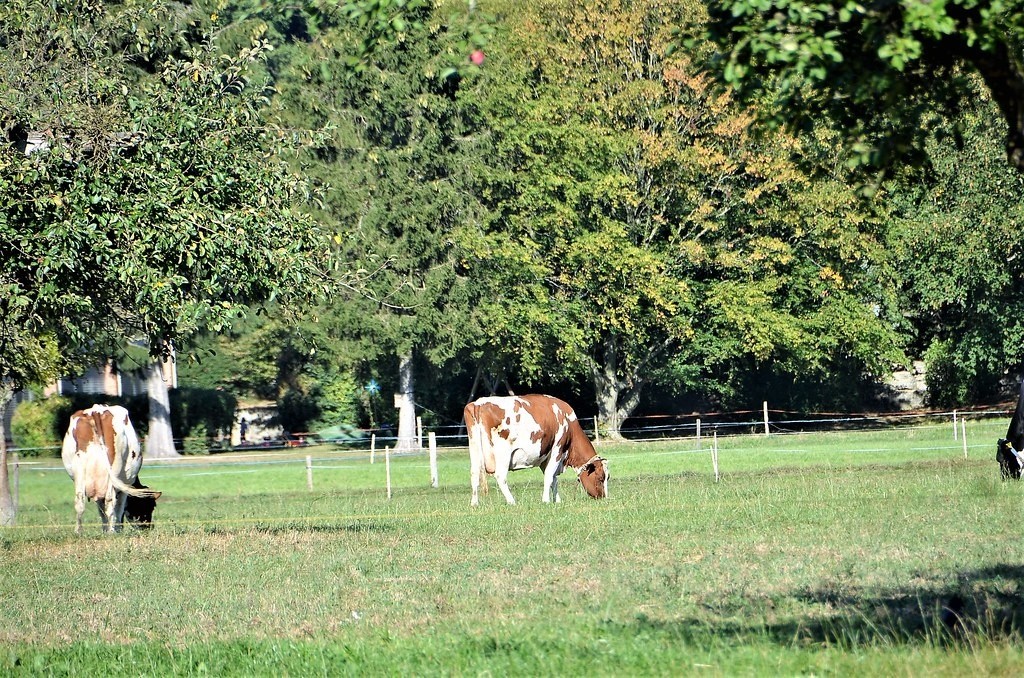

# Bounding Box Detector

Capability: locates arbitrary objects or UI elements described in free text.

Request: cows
[995,377,1024,482]
[464,394,611,506]
[61,402,162,534]
